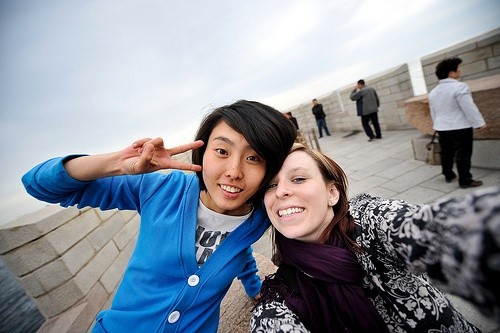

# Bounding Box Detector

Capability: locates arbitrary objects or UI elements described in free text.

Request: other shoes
[368,136,375,142]
[460,179,482,189]
[446,174,457,182]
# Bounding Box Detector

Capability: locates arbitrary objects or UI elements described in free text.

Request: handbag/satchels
[426,131,443,165]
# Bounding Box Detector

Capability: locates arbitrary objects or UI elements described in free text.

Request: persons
[312,99,332,138]
[21,100,298,333]
[427,56,488,188]
[250,144,500,333]
[285,111,299,129]
[350,79,382,142]
